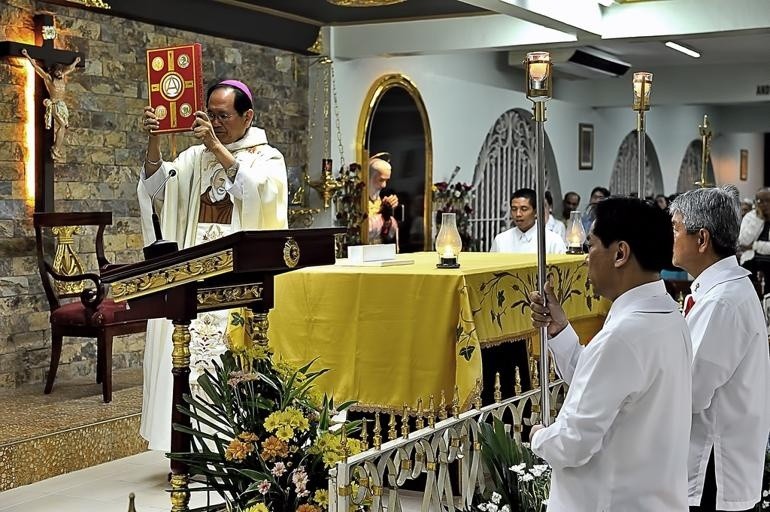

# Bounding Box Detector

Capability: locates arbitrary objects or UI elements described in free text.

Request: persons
[487,187,568,254]
[363,159,402,254]
[131,76,290,490]
[525,185,770,306]
[664,183,770,511]
[523,192,696,511]
[22,47,83,162]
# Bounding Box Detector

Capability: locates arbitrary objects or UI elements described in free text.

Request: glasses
[206,112,238,122]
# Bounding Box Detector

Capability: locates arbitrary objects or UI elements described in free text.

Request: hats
[217,80,252,102]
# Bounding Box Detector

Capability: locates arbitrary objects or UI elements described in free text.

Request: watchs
[223,161,241,178]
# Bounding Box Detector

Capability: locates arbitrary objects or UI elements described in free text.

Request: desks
[269,248,614,496]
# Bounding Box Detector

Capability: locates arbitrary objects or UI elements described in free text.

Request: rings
[144,118,149,125]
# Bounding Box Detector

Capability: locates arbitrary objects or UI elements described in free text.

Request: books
[142,42,208,135]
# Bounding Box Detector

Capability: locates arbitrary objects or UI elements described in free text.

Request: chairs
[31,211,148,404]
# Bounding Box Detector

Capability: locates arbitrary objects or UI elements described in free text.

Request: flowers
[164,313,375,511]
[431,165,486,252]
[440,412,553,511]
[334,162,368,259]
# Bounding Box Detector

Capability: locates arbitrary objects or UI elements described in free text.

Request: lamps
[565,212,587,256]
[435,213,463,269]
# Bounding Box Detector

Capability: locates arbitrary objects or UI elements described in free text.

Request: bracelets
[145,149,163,166]
